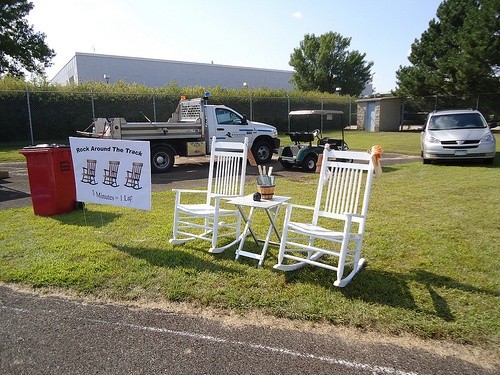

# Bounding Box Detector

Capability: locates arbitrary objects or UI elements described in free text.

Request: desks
[226,194,291,268]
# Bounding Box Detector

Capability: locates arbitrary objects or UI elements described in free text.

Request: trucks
[90,96,278,174]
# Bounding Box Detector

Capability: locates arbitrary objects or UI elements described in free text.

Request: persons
[488,114,497,129]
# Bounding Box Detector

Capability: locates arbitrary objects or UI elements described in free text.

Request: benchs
[290,133,314,155]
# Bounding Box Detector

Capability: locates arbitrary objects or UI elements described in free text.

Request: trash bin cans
[16,141,80,217]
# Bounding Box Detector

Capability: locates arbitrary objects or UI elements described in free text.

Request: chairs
[170,136,252,254]
[273,144,381,287]
[124,162,143,190]
[103,161,120,187]
[81,159,99,186]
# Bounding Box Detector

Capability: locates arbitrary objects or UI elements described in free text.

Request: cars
[417,108,496,166]
